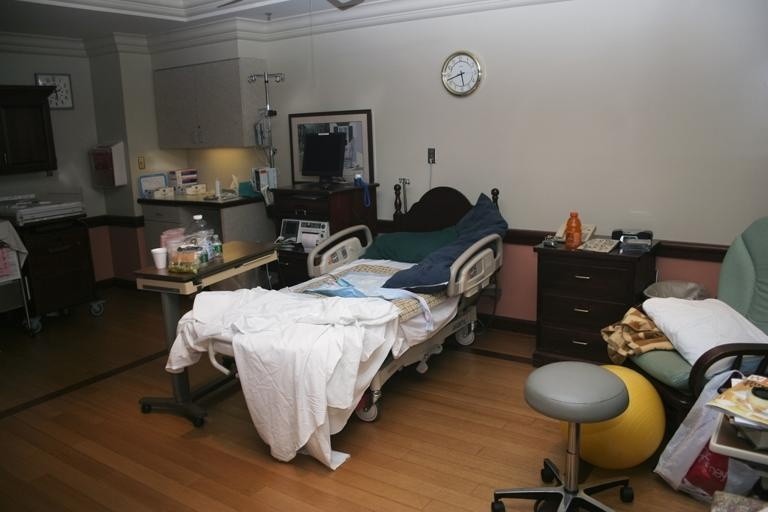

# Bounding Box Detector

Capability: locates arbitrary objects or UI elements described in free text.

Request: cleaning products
[0,189,109,338]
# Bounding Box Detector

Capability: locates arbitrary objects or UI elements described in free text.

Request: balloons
[441,51,482,96]
[35,72,75,110]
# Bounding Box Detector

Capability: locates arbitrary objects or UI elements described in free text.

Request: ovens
[194,184,505,420]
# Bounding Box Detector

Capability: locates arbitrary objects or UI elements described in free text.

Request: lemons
[161,227,185,264]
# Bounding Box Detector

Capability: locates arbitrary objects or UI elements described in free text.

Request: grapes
[532,233,661,368]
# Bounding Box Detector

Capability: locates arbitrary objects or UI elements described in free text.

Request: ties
[302,132,347,189]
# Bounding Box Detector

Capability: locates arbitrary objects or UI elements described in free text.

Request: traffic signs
[428,147,436,164]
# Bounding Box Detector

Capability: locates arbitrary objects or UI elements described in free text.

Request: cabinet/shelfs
[189,213,208,240]
[199,244,210,266]
[566,212,583,249]
[210,233,222,259]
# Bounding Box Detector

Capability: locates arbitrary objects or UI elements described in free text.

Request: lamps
[629,215,768,448]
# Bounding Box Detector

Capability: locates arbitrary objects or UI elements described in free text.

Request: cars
[151,249,166,270]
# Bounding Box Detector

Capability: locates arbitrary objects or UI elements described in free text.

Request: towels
[643,281,700,300]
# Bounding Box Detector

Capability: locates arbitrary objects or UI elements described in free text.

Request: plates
[153,56,272,148]
[0,85,58,177]
[269,183,379,250]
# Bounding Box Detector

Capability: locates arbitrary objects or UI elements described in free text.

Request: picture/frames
[137,180,275,267]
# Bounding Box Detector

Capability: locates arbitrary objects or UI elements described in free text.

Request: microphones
[492,361,634,512]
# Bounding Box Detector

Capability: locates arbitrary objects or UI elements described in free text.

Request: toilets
[545,221,597,244]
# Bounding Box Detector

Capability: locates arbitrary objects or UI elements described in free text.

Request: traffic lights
[642,297,768,382]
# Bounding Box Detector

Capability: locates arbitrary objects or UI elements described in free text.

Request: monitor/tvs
[703,374,768,431]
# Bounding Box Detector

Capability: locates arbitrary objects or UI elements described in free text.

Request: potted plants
[288,109,375,186]
